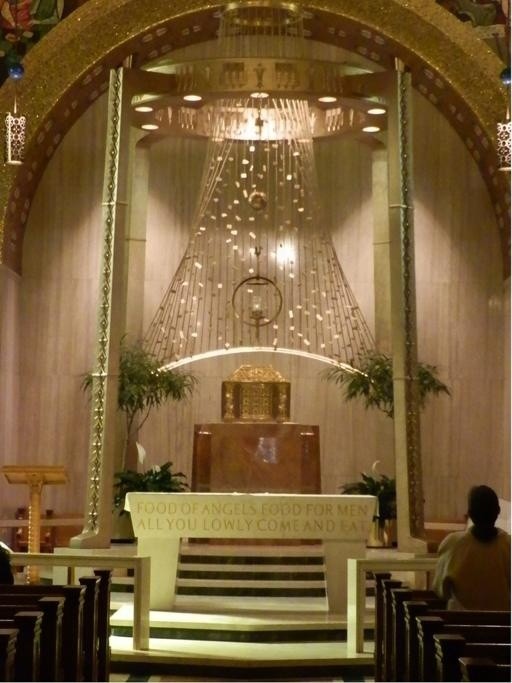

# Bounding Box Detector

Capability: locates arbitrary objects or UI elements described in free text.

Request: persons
[432,482,511,611]
[0,548,14,586]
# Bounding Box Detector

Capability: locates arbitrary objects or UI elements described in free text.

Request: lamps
[4,63,28,166]
[494,65,510,174]
[108,0,391,394]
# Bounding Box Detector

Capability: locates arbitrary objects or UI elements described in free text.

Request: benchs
[0,563,115,681]
[368,564,511,682]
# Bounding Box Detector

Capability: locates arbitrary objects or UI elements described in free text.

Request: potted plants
[336,456,397,549]
